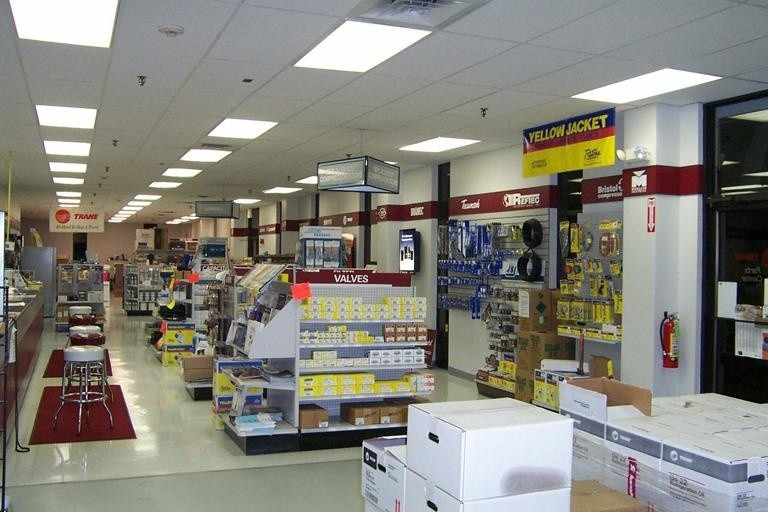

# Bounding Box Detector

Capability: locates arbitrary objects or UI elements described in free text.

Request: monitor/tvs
[399,228,421,274]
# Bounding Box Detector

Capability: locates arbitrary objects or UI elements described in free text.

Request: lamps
[616,144,652,164]
[316,129,400,194]
[194,182,241,219]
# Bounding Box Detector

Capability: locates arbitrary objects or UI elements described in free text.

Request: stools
[51,305,114,435]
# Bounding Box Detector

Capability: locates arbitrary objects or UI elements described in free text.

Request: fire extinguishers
[660,311,680,369]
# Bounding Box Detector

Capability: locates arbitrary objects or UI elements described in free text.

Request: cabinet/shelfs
[122,265,433,457]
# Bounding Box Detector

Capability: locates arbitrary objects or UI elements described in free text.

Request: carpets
[27,349,136,445]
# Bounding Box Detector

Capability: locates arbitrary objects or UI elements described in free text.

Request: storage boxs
[360,287,768,512]
